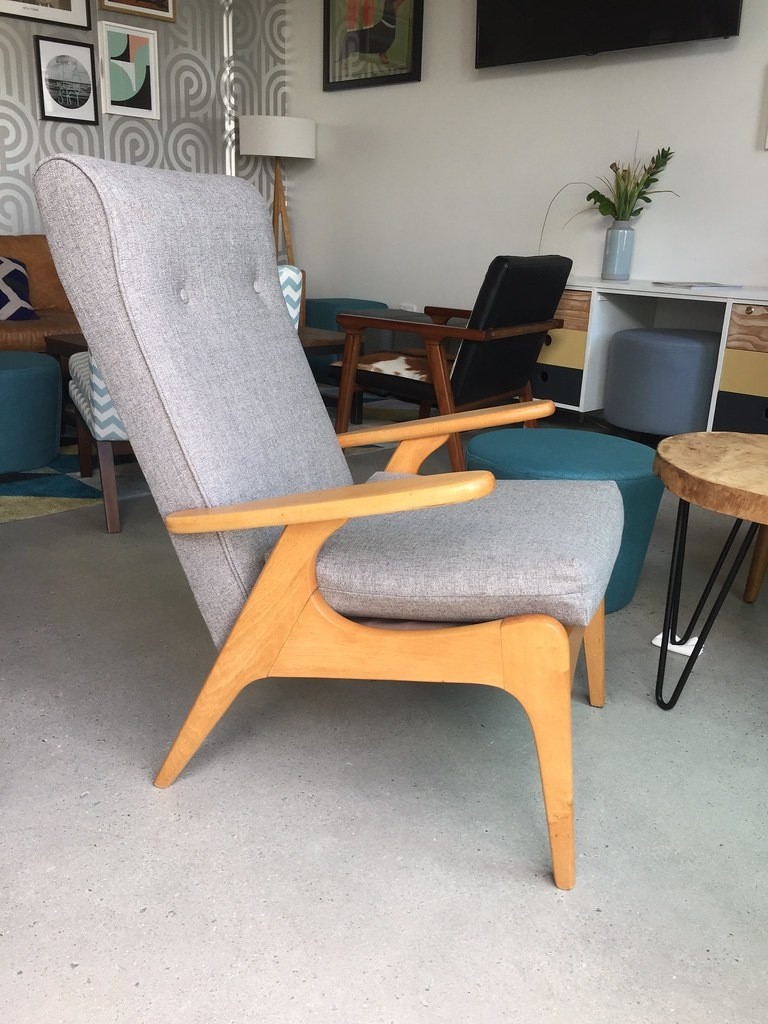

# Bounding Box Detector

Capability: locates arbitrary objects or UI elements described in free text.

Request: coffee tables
[43,326,366,426]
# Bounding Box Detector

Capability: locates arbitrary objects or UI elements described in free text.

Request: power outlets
[399,304,417,312]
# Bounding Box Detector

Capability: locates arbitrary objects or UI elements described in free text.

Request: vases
[601,220,635,281]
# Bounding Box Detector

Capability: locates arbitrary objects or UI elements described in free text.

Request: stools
[463,427,664,615]
[305,298,393,389]
[0,350,63,475]
[603,328,721,436]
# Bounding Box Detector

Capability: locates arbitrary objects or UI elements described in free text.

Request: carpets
[0,381,441,523]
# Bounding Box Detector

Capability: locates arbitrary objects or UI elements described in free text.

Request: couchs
[0,234,82,353]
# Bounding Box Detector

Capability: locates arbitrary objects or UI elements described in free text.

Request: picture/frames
[322,0,424,92]
[0,0,92,31]
[98,0,177,23]
[33,34,100,126]
[97,20,161,121]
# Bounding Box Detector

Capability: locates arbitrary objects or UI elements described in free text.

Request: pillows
[0,256,40,321]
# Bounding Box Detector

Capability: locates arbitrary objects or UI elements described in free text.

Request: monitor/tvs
[475,0,743,69]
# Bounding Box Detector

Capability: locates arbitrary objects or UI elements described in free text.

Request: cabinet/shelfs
[513,277,768,436]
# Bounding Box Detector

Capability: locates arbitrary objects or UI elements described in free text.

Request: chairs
[32,153,625,890]
[335,255,573,472]
[67,265,303,533]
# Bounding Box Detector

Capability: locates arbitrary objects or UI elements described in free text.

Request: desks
[653,432,768,710]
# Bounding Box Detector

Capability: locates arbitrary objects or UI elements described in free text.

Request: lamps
[238,115,317,265]
